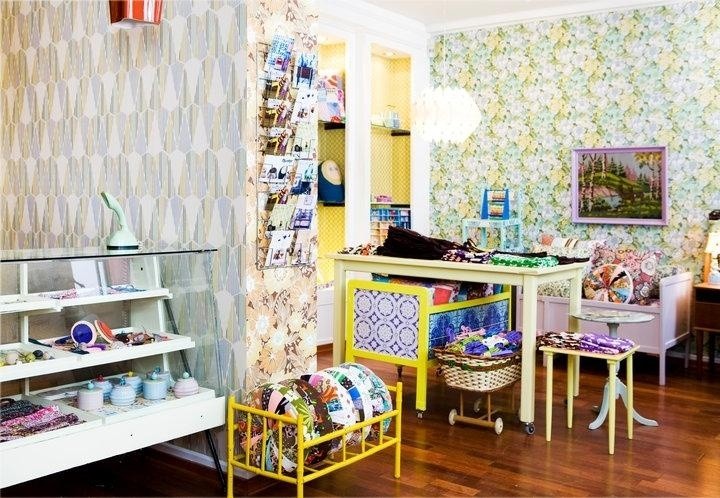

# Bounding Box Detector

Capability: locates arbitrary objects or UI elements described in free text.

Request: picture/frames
[571,146,668,225]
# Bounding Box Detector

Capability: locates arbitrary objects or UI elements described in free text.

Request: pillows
[531,235,664,306]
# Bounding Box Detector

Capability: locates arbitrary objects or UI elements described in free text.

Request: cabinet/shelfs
[0,248,227,498]
[693,282,720,379]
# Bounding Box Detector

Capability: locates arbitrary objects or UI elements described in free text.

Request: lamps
[705,232,720,285]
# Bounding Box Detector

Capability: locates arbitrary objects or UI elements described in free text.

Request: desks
[333,253,591,435]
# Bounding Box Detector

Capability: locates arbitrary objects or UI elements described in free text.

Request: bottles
[383,104,401,128]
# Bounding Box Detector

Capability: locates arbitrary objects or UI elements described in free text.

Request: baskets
[432,346,522,393]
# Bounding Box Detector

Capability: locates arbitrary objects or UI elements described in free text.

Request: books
[260,34,317,267]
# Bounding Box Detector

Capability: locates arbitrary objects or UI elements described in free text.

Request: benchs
[515,265,692,385]
